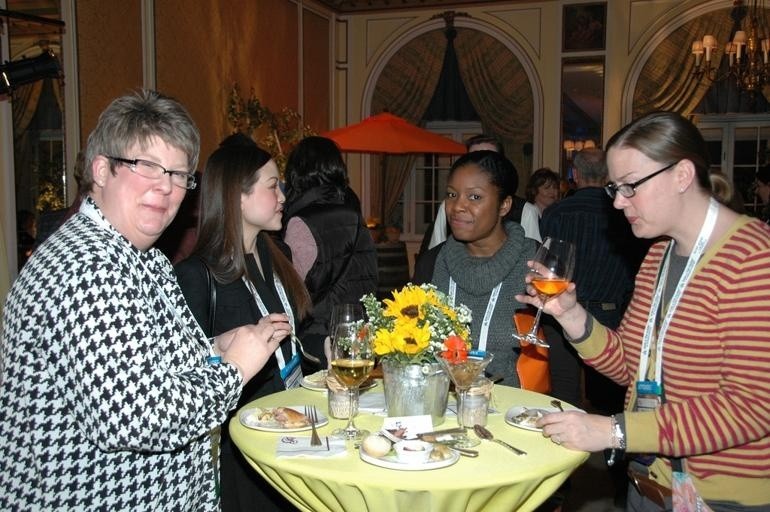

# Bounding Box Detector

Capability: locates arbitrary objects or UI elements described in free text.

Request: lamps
[689,0,769,96]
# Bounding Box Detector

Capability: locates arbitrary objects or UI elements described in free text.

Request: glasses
[603,160,680,200]
[106,155,197,191]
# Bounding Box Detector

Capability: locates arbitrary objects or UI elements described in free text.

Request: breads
[362,434,391,457]
[276,406,308,428]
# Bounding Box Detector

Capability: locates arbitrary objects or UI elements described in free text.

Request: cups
[330,303,365,351]
[454,379,493,428]
[328,369,360,419]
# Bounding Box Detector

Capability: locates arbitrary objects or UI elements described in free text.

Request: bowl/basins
[394,438,434,461]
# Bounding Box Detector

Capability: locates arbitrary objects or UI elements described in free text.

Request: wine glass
[329,323,376,441]
[434,349,494,448]
[509,236,577,348]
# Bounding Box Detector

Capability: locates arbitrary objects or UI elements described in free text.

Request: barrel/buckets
[383,358,450,426]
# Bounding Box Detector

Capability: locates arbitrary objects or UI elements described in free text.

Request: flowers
[333,281,474,366]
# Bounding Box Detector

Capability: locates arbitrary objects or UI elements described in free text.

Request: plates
[504,405,587,432]
[300,375,377,391]
[239,404,329,432]
[358,442,461,470]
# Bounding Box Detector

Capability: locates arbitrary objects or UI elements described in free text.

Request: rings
[555,433,561,444]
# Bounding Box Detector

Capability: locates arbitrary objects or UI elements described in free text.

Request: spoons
[287,330,320,363]
[474,424,527,456]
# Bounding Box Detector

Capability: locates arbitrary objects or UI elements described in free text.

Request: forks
[380,429,479,458]
[304,404,322,446]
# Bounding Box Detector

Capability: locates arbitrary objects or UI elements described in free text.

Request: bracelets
[607,412,623,468]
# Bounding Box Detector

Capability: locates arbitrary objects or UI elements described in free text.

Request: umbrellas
[317,110,467,229]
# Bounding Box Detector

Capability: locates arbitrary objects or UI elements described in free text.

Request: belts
[626,465,674,511]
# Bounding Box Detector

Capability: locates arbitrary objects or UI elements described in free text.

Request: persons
[173,145,333,511]
[0,88,277,512]
[156,170,203,265]
[413,150,582,408]
[282,136,378,375]
[426,134,543,253]
[35,150,87,255]
[513,110,769,510]
